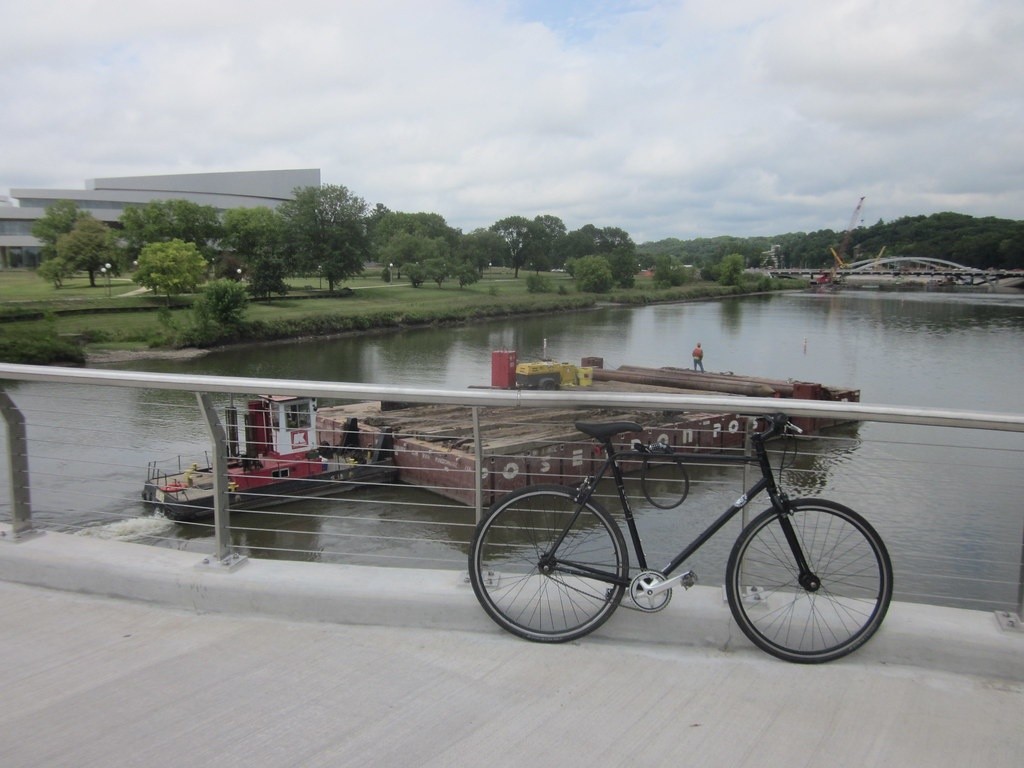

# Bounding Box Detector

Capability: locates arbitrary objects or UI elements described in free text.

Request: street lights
[101,262,112,297]
[318,265,322,289]
[488,262,492,280]
[390,262,393,285]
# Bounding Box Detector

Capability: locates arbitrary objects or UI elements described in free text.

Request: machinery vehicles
[830,196,865,276]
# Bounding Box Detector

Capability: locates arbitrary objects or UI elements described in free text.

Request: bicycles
[469,412,894,666]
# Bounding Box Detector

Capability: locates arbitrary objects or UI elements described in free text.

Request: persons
[692,341,706,373]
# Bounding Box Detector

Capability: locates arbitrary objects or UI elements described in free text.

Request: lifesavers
[160,483,187,492]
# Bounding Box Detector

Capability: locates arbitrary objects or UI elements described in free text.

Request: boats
[140,394,397,524]
